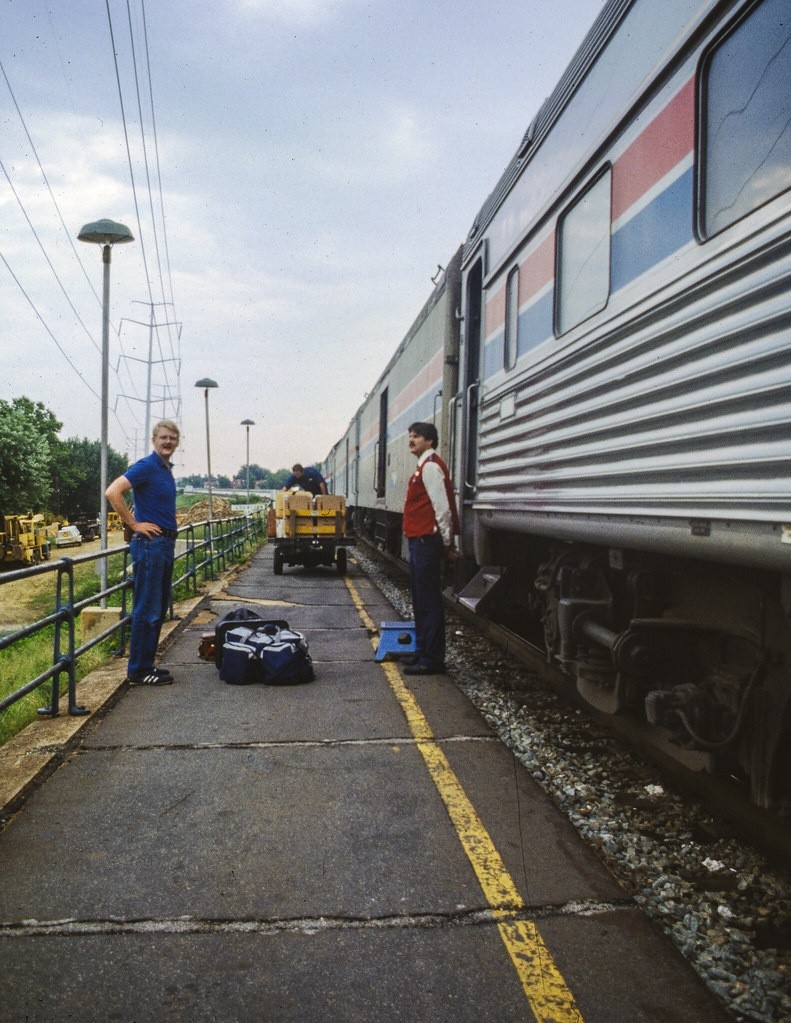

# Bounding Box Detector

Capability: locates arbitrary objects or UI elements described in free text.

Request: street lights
[240,419,255,515]
[194,377,217,555]
[78,216,134,657]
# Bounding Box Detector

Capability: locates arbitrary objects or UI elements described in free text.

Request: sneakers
[130,668,174,686]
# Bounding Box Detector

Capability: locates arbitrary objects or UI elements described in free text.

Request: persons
[400,421,463,673]
[102,421,180,686]
[281,463,330,496]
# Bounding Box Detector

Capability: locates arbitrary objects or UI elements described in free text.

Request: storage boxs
[275,491,347,538]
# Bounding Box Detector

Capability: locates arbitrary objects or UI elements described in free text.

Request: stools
[374,621,417,662]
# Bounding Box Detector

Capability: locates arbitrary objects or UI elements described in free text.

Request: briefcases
[214,619,291,671]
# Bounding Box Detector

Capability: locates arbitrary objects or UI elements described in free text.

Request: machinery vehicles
[106,512,124,532]
[77,516,101,541]
[0,512,51,567]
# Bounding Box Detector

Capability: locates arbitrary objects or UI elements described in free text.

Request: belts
[133,528,178,539]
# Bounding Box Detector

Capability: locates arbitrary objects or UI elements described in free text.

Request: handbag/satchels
[219,624,314,686]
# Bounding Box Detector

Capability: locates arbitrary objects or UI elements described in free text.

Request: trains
[325,3,791,869]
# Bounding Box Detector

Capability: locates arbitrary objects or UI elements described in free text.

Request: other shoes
[399,656,446,676]
